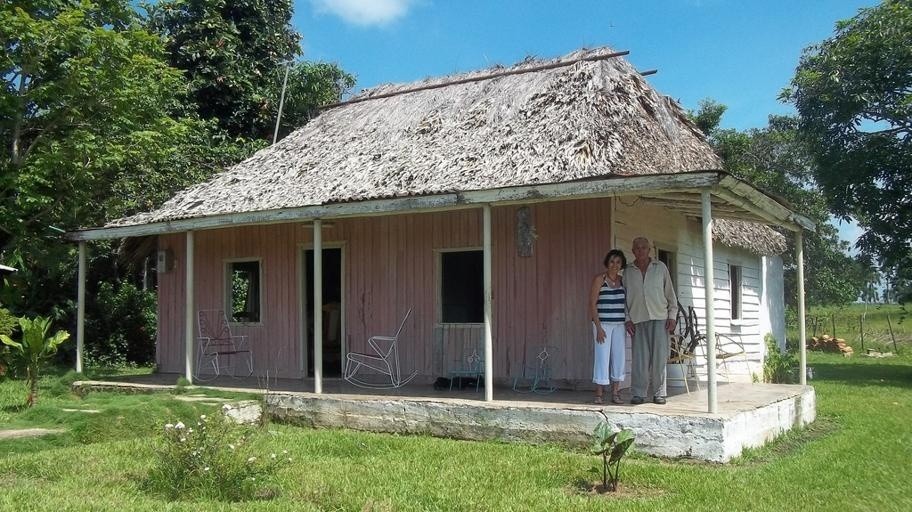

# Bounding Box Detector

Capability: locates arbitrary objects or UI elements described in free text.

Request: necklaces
[605,271,621,287]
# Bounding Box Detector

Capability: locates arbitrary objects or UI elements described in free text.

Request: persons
[589,249,627,404]
[622,237,679,405]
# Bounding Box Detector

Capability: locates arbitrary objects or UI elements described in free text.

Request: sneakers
[612,394,624,404]
[654,396,665,404]
[631,396,644,403]
[593,396,603,404]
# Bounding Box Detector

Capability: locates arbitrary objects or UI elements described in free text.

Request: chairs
[510,344,560,395]
[344,304,419,390]
[190,308,255,382]
[447,348,485,394]
[667,297,762,404]
